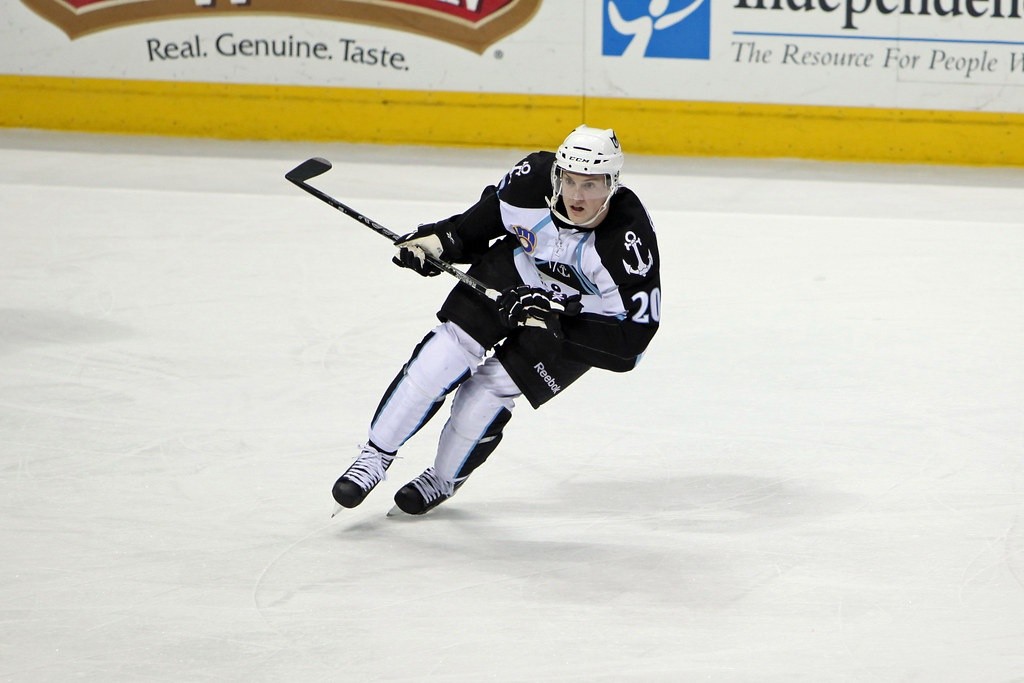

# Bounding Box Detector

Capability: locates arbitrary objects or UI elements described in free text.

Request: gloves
[497,284,566,342]
[392,218,468,277]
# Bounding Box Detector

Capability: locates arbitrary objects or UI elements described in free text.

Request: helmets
[551,125,625,190]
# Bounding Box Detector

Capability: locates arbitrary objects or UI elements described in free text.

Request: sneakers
[388,466,471,516]
[330,440,406,519]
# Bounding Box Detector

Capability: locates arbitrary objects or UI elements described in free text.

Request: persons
[329,124,663,520]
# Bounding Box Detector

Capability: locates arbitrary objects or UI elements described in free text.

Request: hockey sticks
[282,156,502,306]
